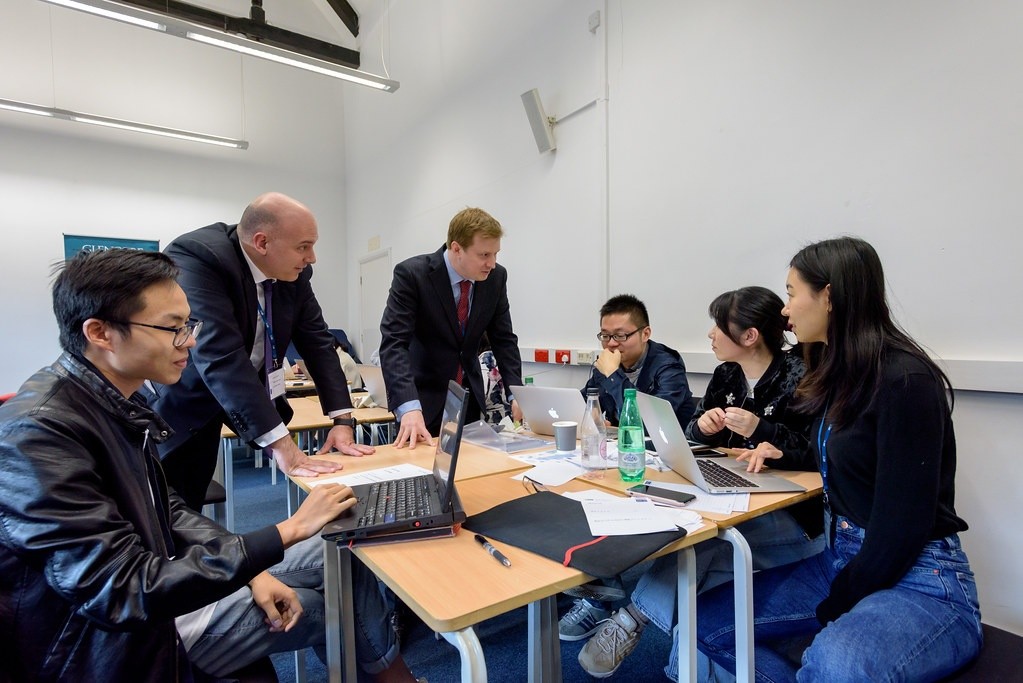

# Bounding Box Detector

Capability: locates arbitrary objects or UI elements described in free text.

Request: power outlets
[556,350,569,364]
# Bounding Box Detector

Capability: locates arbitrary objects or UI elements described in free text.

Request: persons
[138,192,375,515]
[697,237,983,683]
[377,208,524,450]
[303,337,363,447]
[558,294,693,640]
[0,247,416,683]
[578,286,825,683]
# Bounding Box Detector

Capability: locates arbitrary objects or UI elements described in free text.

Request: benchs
[762,624,1023,683]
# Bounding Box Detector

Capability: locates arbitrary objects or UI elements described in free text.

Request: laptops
[635,391,809,492]
[509,386,620,440]
[356,364,389,409]
[321,380,469,541]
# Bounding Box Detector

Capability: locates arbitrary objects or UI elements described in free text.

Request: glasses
[96,311,204,348]
[597,324,651,343]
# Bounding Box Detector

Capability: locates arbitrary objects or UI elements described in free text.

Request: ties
[454,280,472,387]
[260,276,275,460]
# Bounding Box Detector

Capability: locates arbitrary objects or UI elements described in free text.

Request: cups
[552,421,578,454]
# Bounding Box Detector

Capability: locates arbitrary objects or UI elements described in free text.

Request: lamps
[0,0,249,151]
[40,0,400,93]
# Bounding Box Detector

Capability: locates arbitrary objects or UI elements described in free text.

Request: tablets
[645,439,710,456]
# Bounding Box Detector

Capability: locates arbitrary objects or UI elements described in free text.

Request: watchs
[334,417,357,428]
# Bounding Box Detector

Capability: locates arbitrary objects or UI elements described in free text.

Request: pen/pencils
[475,535,511,568]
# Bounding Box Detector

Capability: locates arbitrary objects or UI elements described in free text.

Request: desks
[215,378,826,682]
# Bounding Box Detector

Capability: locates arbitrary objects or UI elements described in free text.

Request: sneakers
[558,597,615,641]
[578,608,642,678]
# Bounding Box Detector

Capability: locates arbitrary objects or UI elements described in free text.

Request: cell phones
[693,448,728,458]
[626,484,697,507]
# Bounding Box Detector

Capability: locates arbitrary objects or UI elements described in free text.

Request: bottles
[618,389,646,480]
[580,387,608,480]
[523,377,536,431]
